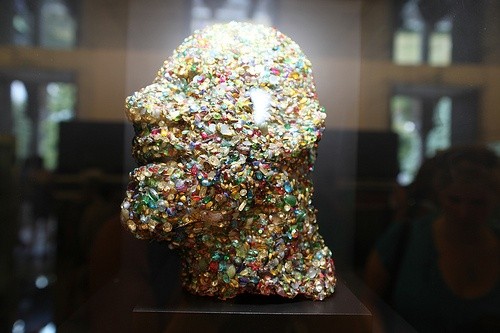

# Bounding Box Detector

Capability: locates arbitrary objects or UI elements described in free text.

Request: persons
[15,155,55,244]
[359,145,500,333]
